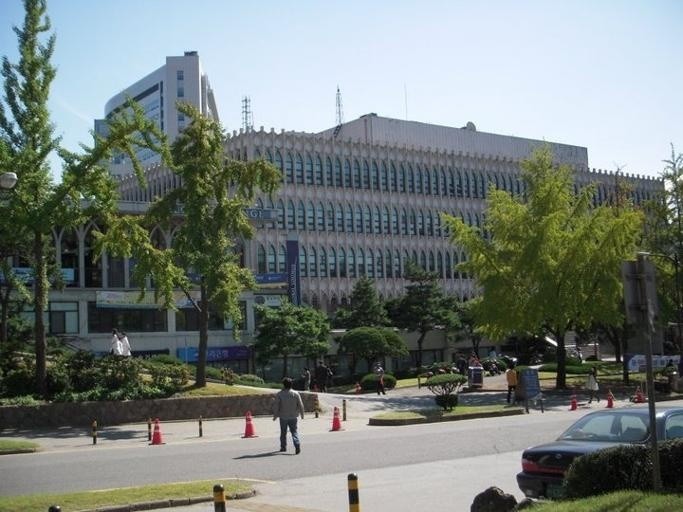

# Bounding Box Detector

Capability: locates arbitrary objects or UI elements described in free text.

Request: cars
[515,405,682,502]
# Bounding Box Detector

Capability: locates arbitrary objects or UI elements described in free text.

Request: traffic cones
[329,406,345,432]
[148,418,166,445]
[240,412,259,438]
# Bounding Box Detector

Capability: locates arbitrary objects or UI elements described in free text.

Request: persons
[506,364,518,404]
[273,378,305,455]
[108,328,132,358]
[588,366,600,403]
[372,362,385,394]
[301,361,332,392]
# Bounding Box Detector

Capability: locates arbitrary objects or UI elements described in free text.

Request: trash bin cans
[468,366,483,387]
